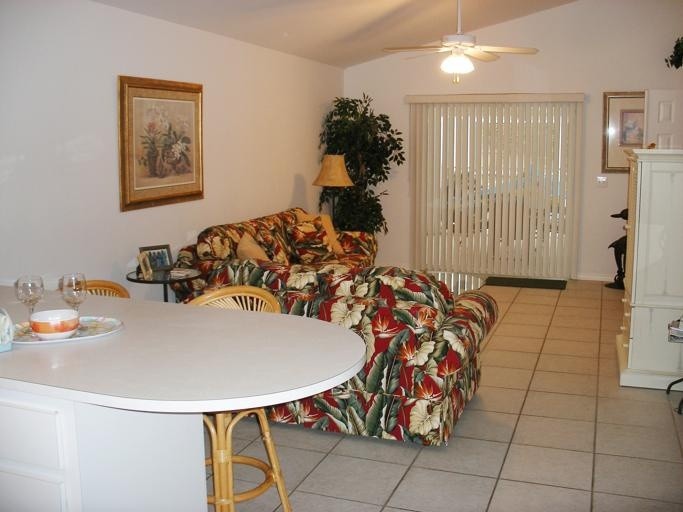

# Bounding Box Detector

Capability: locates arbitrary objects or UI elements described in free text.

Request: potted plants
[116,74,204,213]
[139,244,173,272]
[137,252,152,280]
[599,89,646,174]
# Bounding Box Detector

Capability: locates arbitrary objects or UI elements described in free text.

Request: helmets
[12,315,123,343]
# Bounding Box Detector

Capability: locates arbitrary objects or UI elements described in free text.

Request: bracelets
[29,309,78,339]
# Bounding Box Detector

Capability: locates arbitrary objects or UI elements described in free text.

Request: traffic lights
[212,213,347,267]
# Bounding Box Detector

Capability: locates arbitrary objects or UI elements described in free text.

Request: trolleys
[381,1,540,63]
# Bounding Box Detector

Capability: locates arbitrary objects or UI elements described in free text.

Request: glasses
[311,153,354,226]
[438,48,474,85]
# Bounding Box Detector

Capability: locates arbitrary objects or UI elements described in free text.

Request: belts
[61,272,88,332]
[14,275,44,335]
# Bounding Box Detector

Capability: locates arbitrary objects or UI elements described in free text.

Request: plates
[614,145,683,393]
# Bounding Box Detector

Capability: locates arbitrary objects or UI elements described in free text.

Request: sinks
[169,207,499,446]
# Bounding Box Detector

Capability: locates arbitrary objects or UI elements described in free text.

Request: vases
[135,105,192,177]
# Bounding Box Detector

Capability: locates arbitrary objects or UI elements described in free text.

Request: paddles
[481,276,568,290]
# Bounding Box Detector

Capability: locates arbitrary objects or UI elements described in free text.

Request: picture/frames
[1,283,367,512]
[424,271,485,296]
[126,265,202,303]
[665,320,682,416]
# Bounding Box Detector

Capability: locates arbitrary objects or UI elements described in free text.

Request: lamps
[183,284,290,512]
[56,279,130,300]
